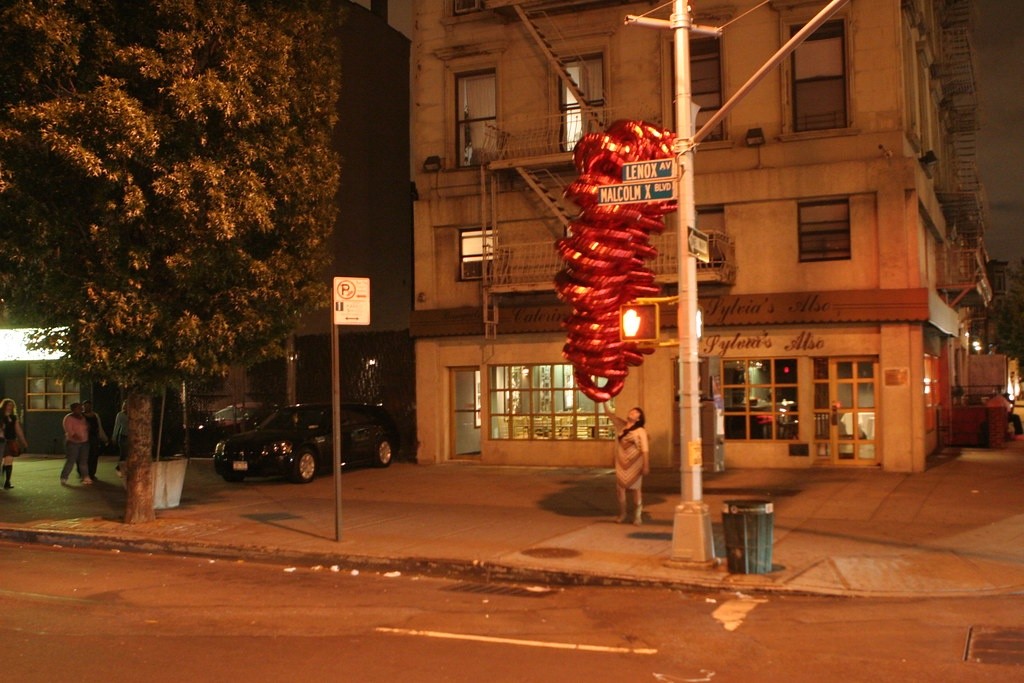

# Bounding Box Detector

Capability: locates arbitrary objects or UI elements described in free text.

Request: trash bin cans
[721,499,775,576]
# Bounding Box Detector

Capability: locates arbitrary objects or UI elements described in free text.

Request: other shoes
[82,475,93,484]
[90,474,97,480]
[60,478,67,485]
[116,468,125,477]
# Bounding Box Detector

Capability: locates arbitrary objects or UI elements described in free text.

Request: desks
[840,412,874,438]
[865,416,874,440]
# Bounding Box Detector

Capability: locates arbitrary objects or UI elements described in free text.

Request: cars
[212,400,402,484]
[194,401,284,456]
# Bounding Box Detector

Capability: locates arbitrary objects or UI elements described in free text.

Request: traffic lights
[620,303,659,344]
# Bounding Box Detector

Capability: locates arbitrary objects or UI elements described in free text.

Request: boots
[616,500,627,523]
[3,465,14,489]
[633,503,643,527]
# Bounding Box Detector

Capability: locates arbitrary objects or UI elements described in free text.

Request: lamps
[746,128,766,148]
[424,156,441,172]
[919,150,939,166]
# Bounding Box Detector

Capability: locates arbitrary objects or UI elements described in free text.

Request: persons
[60,400,109,485]
[0,399,28,490]
[112,399,128,472]
[1004,393,1024,436]
[605,399,649,525]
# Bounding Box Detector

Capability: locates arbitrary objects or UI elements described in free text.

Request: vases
[118,456,188,510]
